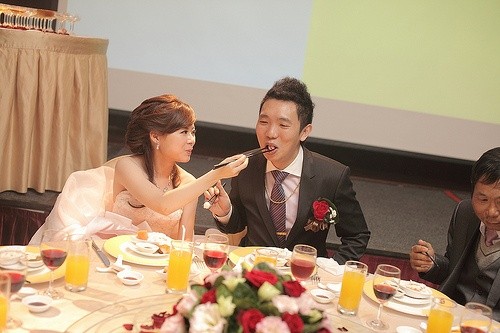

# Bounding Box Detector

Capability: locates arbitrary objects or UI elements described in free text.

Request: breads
[136,231,149,240]
[158,244,171,254]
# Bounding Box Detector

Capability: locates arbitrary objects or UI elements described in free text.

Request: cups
[165,240,193,295]
[291,244,317,283]
[203,234,230,272]
[0,271,11,333]
[65,234,92,292]
[338,260,368,316]
[425,297,492,333]
[255,248,279,266]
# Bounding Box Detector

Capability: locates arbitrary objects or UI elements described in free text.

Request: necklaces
[150,170,173,192]
[264,175,302,204]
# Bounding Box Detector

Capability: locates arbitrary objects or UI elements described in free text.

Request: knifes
[92,240,111,267]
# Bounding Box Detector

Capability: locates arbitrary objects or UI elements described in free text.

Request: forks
[193,256,206,270]
[311,265,320,285]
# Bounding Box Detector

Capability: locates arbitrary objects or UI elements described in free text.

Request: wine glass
[41,228,70,299]
[366,264,401,330]
[0,3,78,36]
[0,249,28,329]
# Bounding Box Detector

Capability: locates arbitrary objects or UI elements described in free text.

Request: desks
[0,27,108,193]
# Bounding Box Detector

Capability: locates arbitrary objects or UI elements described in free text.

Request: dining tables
[0,242,500,333]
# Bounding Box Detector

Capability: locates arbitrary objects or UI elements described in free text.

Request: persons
[203,76,373,265]
[111,93,250,243]
[408,146,500,323]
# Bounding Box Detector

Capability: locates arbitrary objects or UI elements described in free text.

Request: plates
[16,287,53,313]
[104,232,175,266]
[117,270,144,285]
[310,288,336,303]
[396,322,427,333]
[363,279,455,316]
[0,245,66,283]
[229,246,293,267]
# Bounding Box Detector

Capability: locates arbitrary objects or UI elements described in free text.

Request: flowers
[141,262,333,333]
[311,197,339,225]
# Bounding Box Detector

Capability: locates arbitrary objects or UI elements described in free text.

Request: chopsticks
[426,252,440,269]
[202,145,276,208]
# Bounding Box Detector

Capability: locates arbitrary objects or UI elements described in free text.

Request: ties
[269,170,289,247]
